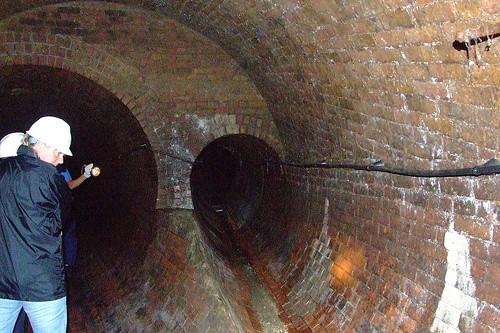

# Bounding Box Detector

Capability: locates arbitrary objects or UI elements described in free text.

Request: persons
[0,116,73,333]
[0,130,100,331]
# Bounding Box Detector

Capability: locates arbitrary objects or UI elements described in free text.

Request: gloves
[82,162,93,179]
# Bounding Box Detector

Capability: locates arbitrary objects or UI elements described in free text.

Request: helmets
[26,115,74,157]
[0,131,27,158]
